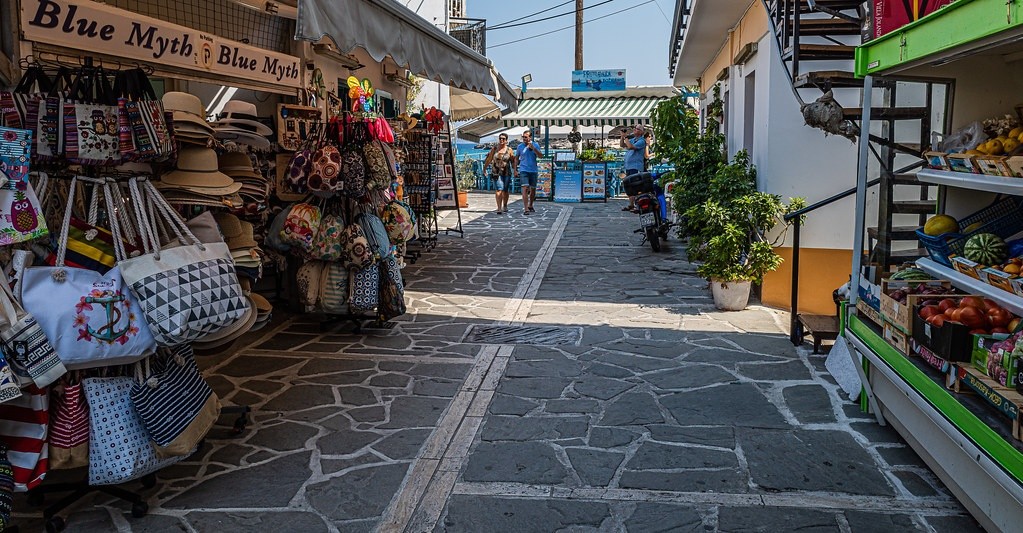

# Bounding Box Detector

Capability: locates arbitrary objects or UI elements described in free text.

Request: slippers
[524,211,529,215]
[528,207,535,212]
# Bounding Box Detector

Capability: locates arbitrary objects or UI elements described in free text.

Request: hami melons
[924,214,984,253]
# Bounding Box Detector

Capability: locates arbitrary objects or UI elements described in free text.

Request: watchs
[624,136,628,139]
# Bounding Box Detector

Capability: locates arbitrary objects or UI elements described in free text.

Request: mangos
[1003,263,1023,277]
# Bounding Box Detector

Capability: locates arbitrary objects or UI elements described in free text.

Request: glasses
[522,135,531,138]
[635,127,642,131]
[499,137,507,140]
[648,136,651,138]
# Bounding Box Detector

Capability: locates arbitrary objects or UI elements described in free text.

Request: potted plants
[648,92,806,311]
[455,155,476,207]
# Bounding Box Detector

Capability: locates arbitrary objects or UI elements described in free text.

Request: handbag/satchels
[264,122,419,324]
[0,63,251,533]
[490,172,499,181]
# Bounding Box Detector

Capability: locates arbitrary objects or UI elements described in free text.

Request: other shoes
[497,210,502,214]
[504,210,508,212]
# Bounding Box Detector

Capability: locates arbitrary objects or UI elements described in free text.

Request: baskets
[915,195,1023,270]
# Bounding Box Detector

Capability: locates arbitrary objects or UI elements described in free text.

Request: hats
[113,92,273,359]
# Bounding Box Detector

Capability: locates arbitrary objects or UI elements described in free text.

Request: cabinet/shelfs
[839,0,1023,532]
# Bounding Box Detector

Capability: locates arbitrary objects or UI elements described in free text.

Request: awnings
[295,0,520,113]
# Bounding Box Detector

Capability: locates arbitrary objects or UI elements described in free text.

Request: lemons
[964,125,1023,156]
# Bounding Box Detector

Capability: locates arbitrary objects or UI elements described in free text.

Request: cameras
[621,128,627,132]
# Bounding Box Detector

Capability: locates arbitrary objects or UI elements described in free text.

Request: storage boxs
[915,195,1023,298]
[923,140,1023,178]
[853,268,1023,444]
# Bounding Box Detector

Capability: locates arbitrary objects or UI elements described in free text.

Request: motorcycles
[620,168,673,253]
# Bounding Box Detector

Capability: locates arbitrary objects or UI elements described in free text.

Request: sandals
[621,205,639,212]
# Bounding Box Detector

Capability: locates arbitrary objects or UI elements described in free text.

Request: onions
[888,283,963,308]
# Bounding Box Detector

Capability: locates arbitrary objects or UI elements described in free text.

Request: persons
[483,130,543,215]
[620,123,655,213]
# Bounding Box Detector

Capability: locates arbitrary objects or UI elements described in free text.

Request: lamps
[383,71,415,88]
[311,40,359,67]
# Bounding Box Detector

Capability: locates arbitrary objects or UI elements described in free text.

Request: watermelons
[888,268,929,280]
[963,232,1009,266]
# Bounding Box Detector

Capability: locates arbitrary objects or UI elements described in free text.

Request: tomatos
[919,297,1020,335]
